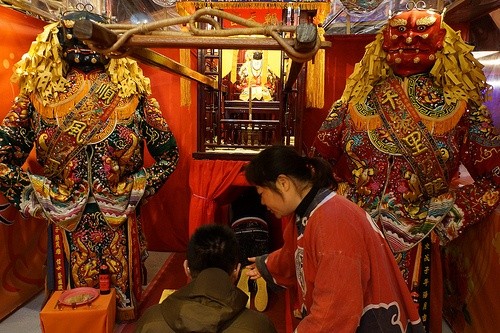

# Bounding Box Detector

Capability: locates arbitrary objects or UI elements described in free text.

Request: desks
[40,288,116,333]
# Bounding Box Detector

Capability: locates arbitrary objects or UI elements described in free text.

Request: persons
[223,188,288,313]
[308,3,500,333]
[127,219,279,333]
[1,4,184,322]
[239,52,276,102]
[245,136,428,332]
